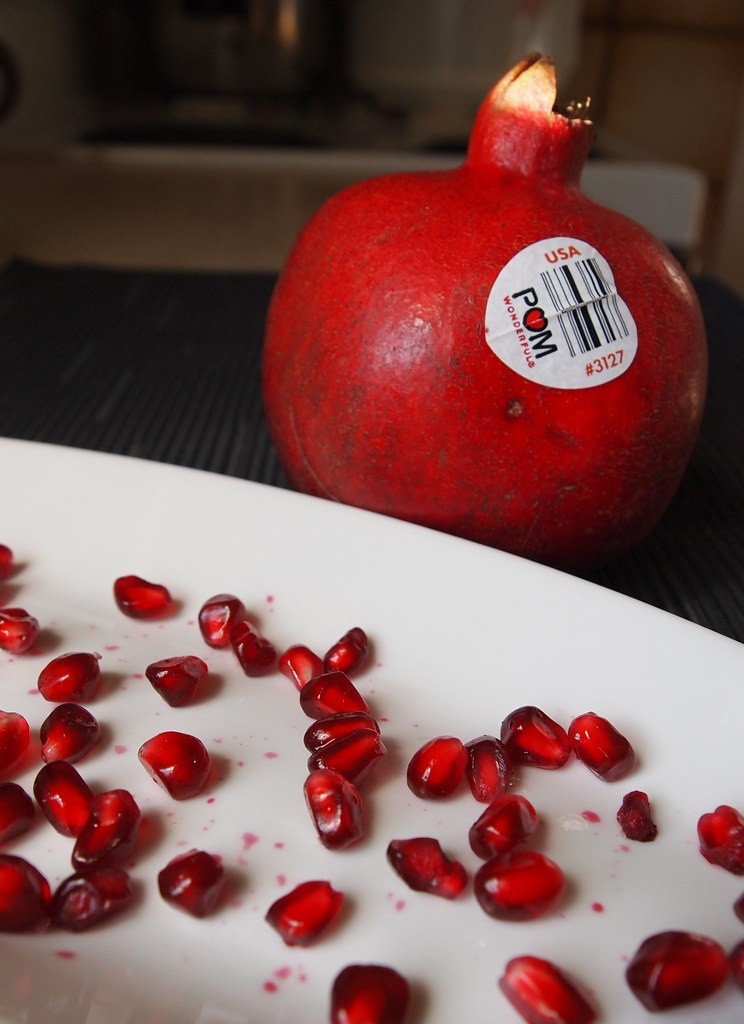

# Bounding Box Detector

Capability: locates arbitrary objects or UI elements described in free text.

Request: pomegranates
[263,50,711,572]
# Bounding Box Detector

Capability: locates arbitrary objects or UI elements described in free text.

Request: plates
[0,437,743,1024]
